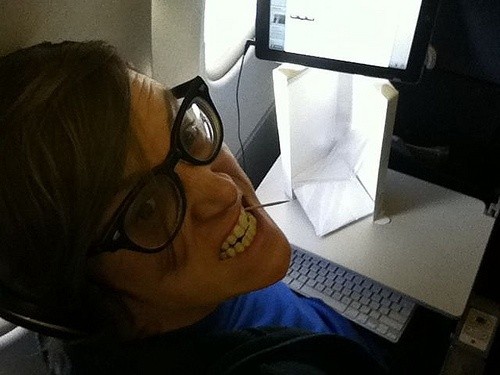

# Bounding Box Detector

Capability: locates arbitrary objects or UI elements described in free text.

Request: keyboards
[280,246,416,343]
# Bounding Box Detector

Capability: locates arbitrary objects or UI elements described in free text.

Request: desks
[255,152,495,319]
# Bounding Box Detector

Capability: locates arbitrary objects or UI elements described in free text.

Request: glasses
[82,75,224,262]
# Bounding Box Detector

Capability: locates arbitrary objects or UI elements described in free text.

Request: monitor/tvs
[251,0,440,86]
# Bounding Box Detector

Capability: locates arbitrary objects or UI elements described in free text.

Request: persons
[0,39,448,375]
